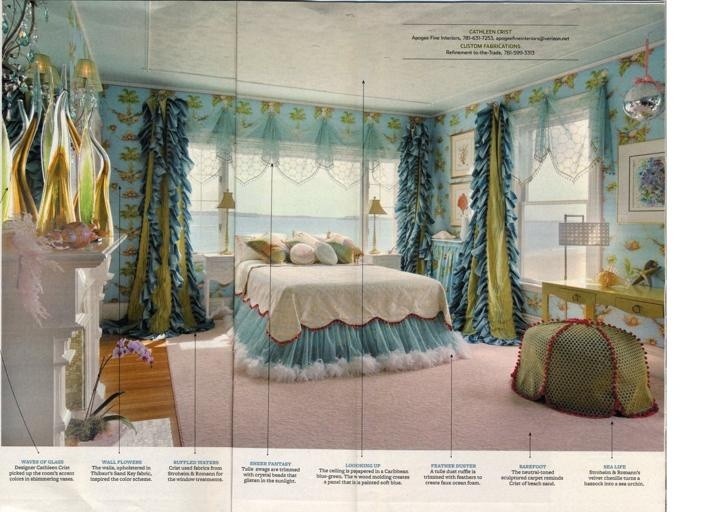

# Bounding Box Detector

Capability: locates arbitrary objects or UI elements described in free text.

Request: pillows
[244,230,364,266]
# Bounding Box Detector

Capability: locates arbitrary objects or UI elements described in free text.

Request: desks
[2,231,127,446]
[543,280,664,325]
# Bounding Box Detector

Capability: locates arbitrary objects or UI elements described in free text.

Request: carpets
[166,316,663,449]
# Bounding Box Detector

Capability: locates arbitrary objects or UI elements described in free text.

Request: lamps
[558,215,611,319]
[217,189,235,255]
[368,196,387,254]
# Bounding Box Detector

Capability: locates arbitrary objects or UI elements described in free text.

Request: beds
[233,234,442,382]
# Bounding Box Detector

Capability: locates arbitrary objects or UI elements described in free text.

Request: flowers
[66,333,154,441]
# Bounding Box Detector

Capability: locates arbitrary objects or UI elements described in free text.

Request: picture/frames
[450,129,478,227]
[617,138,665,226]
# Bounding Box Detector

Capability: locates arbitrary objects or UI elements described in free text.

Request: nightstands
[366,254,402,270]
[203,254,235,319]
